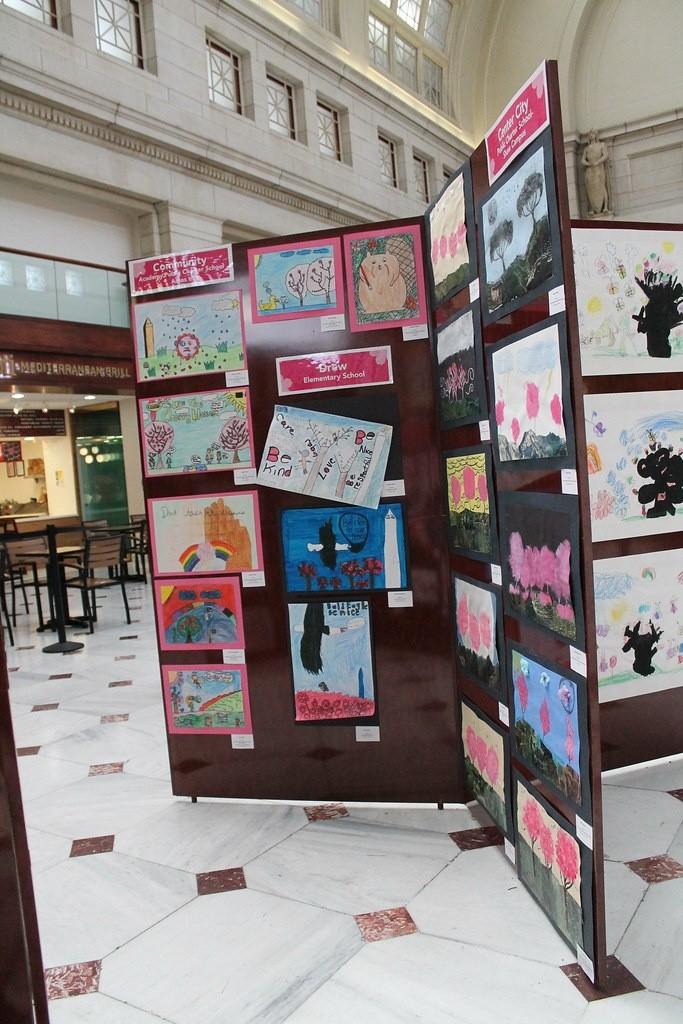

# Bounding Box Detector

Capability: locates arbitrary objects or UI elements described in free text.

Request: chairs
[0,514,147,633]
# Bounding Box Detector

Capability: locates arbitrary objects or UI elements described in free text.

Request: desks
[15,545,89,633]
[90,524,143,581]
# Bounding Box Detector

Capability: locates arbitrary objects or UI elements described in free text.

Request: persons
[581,129,613,215]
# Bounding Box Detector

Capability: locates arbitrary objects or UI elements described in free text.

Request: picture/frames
[511,767,596,982]
[504,637,592,824]
[276,497,412,598]
[484,58,550,187]
[440,444,499,565]
[275,345,394,396]
[422,158,478,312]
[432,299,488,431]
[497,490,585,650]
[154,579,245,651]
[247,237,344,324]
[458,693,514,846]
[147,490,264,577]
[451,571,507,705]
[138,387,255,477]
[162,664,252,735]
[475,127,565,327]
[128,244,234,297]
[285,596,379,727]
[485,312,576,469]
[131,289,248,384]
[344,224,427,332]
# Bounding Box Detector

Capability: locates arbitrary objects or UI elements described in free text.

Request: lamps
[13,405,23,414]
[42,401,48,412]
[69,405,75,413]
[79,443,103,464]
